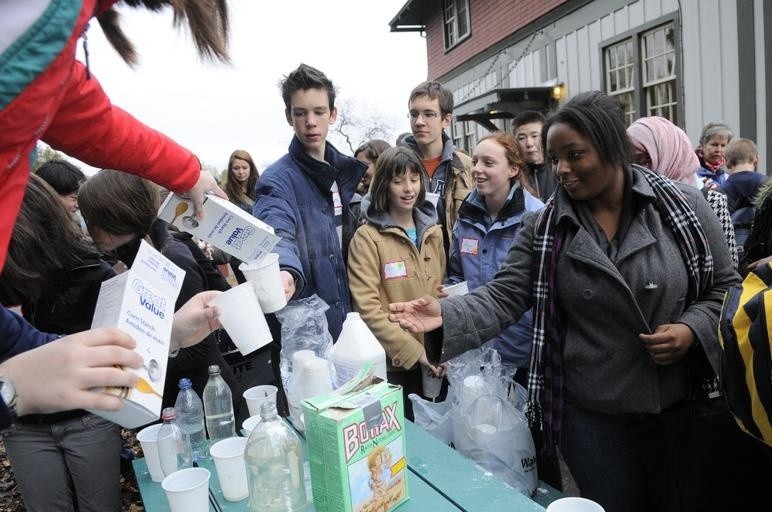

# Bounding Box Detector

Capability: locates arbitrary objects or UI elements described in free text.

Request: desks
[132,412,542,511]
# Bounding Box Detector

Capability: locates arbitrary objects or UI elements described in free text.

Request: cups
[544,496,602,511]
[161,467,211,512]
[441,279,469,297]
[241,415,281,438]
[239,253,288,315]
[243,385,278,416]
[208,280,275,358]
[209,438,248,501]
[136,423,177,483]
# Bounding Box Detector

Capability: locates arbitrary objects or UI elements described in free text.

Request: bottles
[328,313,387,390]
[83,240,186,430]
[202,366,235,441]
[158,409,193,477]
[245,402,308,512]
[156,191,281,268]
[173,380,206,464]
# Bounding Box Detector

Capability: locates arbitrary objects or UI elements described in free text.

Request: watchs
[0,367,23,418]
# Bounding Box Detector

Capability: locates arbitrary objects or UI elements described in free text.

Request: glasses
[407,111,445,120]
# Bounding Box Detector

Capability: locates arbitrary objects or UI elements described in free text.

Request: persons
[385,90,743,512]
[347,145,452,423]
[1,1,230,288]
[77,170,251,438]
[348,139,392,226]
[446,132,547,389]
[2,304,142,434]
[692,122,730,187]
[246,62,364,347]
[394,81,477,264]
[218,149,263,215]
[623,115,740,275]
[0,170,134,511]
[511,107,560,204]
[716,137,768,249]
[34,156,85,220]
